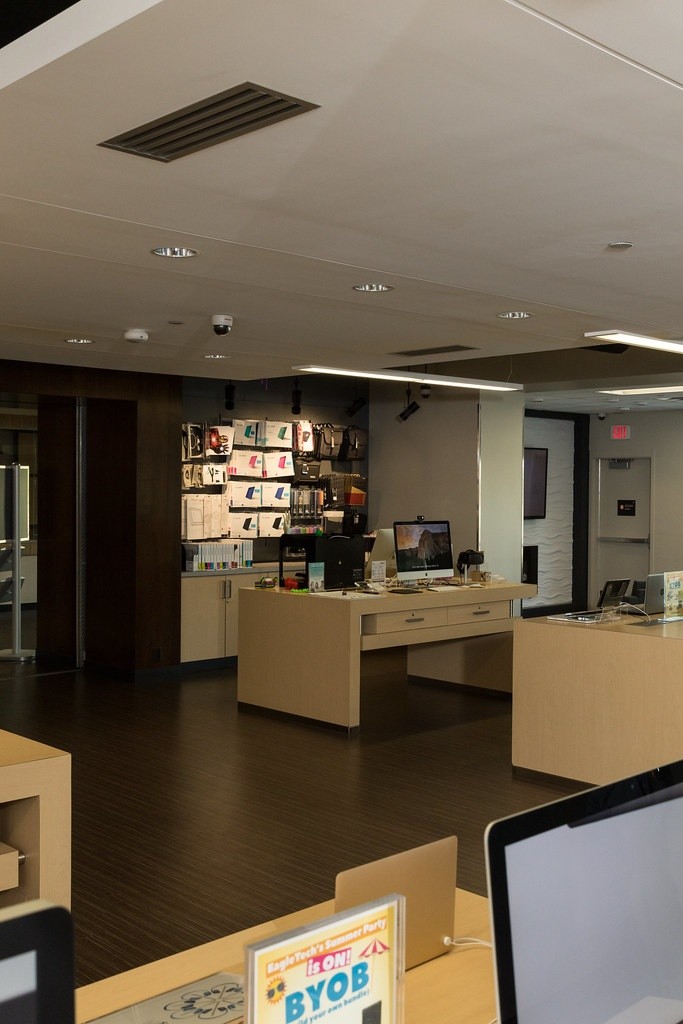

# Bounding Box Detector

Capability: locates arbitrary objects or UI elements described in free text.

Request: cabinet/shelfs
[180,571,298,675]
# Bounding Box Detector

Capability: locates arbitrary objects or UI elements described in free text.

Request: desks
[513,602,683,785]
[74,888,499,1024]
[237,576,539,732]
[0,728,71,923]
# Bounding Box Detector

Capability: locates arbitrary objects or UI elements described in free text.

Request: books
[181,538,254,571]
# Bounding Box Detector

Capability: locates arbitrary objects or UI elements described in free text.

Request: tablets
[596,578,630,607]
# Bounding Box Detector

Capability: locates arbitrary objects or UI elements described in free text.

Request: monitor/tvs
[392,519,454,580]
[482,759,683,1024]
[306,533,365,591]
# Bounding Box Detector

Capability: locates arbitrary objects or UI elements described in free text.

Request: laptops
[617,574,664,614]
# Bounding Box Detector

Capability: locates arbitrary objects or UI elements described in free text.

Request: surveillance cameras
[210,315,234,336]
[420,389,431,399]
[598,414,605,420]
